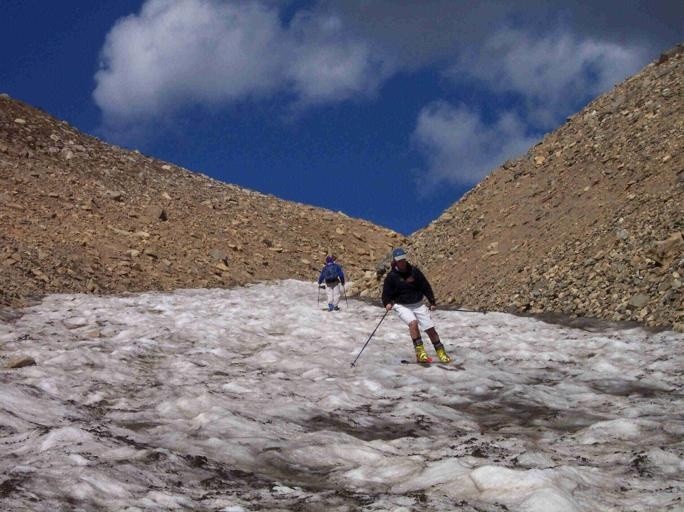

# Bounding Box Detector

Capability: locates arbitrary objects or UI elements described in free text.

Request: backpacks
[324,268,336,281]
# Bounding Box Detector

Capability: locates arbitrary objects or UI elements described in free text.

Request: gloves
[318,280,322,284]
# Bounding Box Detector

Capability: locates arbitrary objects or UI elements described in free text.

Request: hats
[393,248,406,261]
[326,256,332,263]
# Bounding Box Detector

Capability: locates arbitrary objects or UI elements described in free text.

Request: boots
[328,304,340,311]
[415,344,432,362]
[435,344,450,363]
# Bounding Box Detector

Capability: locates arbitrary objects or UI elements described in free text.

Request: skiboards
[401,356,466,367]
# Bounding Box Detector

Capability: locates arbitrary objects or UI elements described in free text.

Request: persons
[382,249,450,363]
[318,256,345,311]
